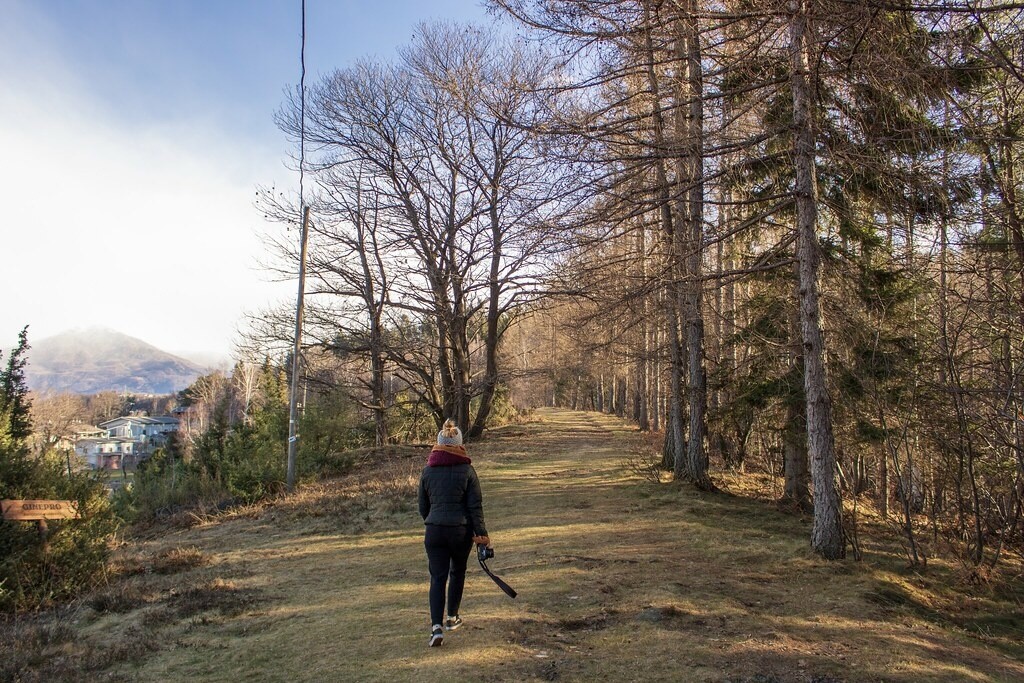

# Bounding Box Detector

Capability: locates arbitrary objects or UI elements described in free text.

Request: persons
[417,418,490,647]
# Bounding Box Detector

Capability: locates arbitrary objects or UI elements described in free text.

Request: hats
[438,419,463,446]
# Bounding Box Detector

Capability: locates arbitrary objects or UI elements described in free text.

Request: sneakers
[445,615,463,630]
[429,628,443,647]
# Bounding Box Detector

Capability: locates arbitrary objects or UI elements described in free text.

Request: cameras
[477,543,494,561]
